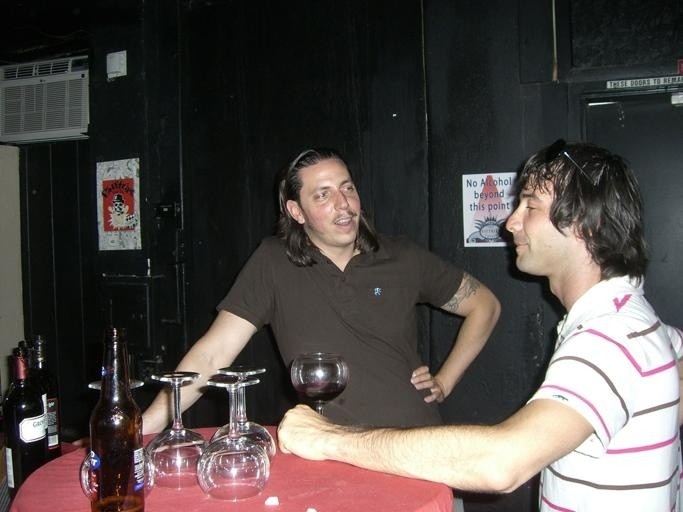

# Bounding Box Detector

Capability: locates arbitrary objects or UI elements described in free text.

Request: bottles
[90,326,145,512]
[4,333,62,502]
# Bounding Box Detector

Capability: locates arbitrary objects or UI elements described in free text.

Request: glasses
[545,137,595,186]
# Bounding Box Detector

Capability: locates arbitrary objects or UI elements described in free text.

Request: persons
[276,137,683,511]
[73,149,503,512]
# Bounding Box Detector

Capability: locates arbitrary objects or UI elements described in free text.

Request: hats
[112,194,124,203]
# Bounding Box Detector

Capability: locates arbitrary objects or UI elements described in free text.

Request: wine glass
[145,370,196,485]
[288,349,351,417]
[197,360,279,501]
